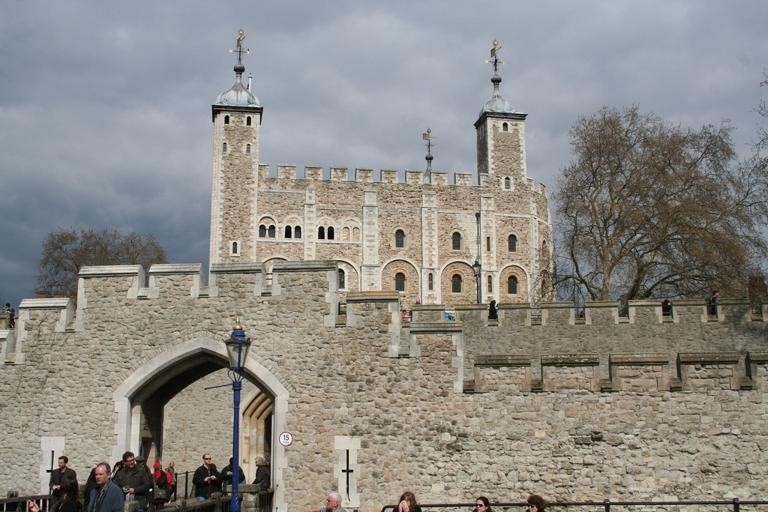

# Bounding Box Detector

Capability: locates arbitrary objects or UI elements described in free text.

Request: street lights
[222,316,252,512]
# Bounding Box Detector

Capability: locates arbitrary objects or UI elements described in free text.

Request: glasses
[528,505,534,508]
[205,458,211,460]
[476,504,484,507]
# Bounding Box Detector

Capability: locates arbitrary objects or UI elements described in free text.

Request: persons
[472,496,495,512]
[27,449,269,512]
[525,494,547,512]
[399,299,455,322]
[707,290,720,315]
[662,299,671,316]
[304,492,347,512]
[392,492,422,512]
[488,300,498,320]
[5,302,16,327]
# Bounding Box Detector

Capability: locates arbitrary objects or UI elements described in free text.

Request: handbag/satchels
[153,486,168,499]
[124,493,140,512]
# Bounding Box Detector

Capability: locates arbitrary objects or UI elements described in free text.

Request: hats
[153,464,162,471]
[135,456,145,461]
